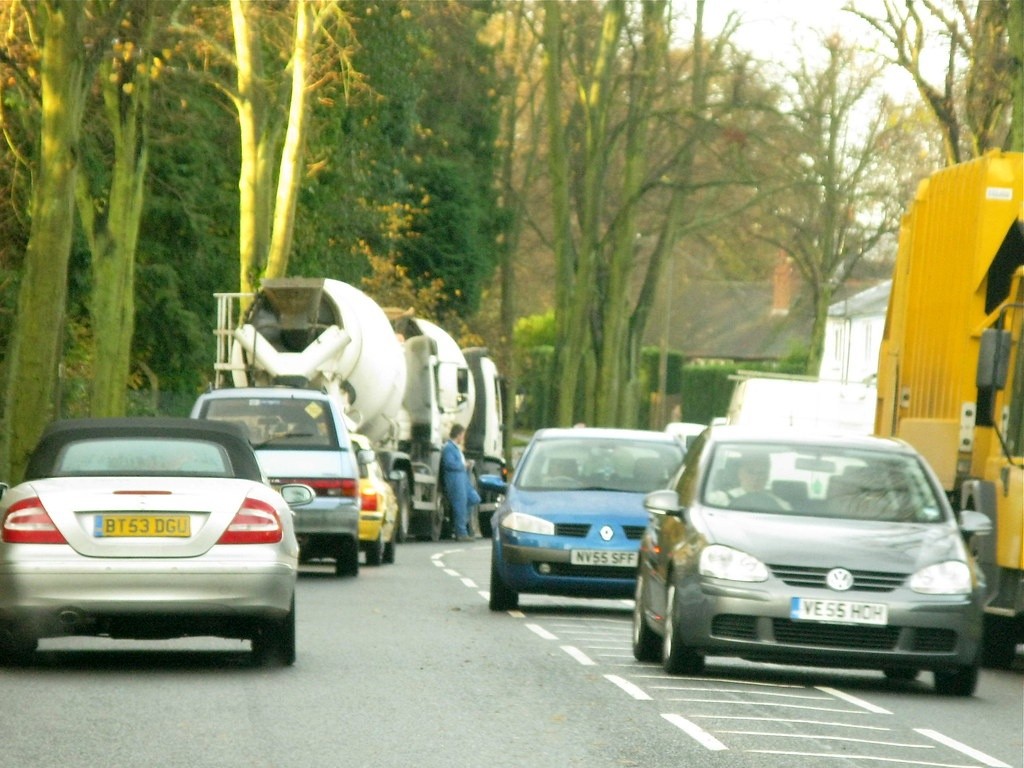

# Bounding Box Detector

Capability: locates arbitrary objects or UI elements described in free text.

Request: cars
[296,423,410,568]
[1,410,317,664]
[480,428,691,612]
[633,423,994,696]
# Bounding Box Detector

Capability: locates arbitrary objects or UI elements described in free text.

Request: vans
[184,381,379,578]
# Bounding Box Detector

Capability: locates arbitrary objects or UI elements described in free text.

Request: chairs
[827,466,887,500]
[772,479,808,511]
[631,458,668,488]
[721,457,740,489]
[548,458,579,481]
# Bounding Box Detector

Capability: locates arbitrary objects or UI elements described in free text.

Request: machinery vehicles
[211,270,504,544]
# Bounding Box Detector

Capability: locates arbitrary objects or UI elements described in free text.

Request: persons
[438,421,481,540]
[709,449,792,513]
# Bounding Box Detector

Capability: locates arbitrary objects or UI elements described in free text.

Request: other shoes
[456,535,474,542]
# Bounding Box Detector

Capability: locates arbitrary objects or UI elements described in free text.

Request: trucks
[869,143,1024,673]
